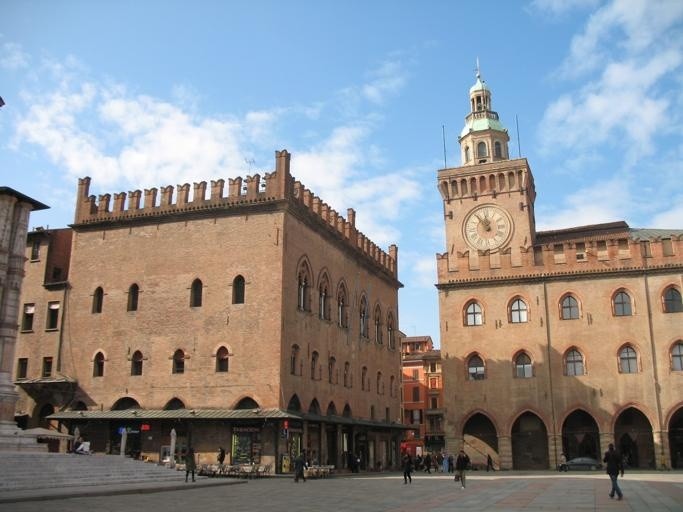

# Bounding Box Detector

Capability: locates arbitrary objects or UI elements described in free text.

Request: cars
[559,456,602,473]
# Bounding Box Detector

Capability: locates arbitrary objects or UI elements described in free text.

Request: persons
[183,446,196,482]
[75,436,83,450]
[293,451,308,481]
[485,453,495,472]
[215,446,225,464]
[401,453,412,484]
[557,451,567,472]
[602,442,625,502]
[415,452,455,474]
[74,435,90,452]
[453,449,471,490]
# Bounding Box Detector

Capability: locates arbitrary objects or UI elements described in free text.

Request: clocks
[462,203,514,254]
